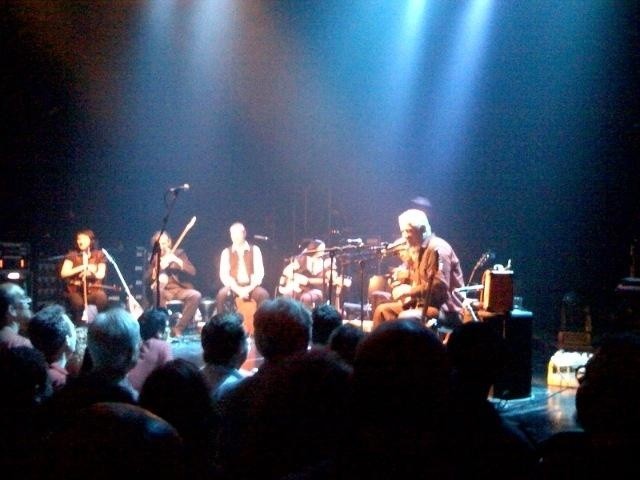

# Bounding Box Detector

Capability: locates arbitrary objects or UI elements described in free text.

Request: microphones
[254,235,269,243]
[346,237,362,245]
[387,239,407,248]
[170,183,191,193]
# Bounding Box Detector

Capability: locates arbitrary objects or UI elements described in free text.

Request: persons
[257,349,355,479]
[540,332,640,479]
[306,305,342,357]
[29,304,77,387]
[368,240,412,306]
[352,319,447,479]
[0,283,33,348]
[62,401,185,478]
[61,229,107,313]
[144,231,202,339]
[199,312,249,400]
[284,240,336,305]
[1,346,59,479]
[58,308,142,410]
[123,310,172,393]
[216,222,269,315]
[138,358,224,466]
[221,298,313,410]
[444,322,541,480]
[373,209,464,328]
[328,323,367,365]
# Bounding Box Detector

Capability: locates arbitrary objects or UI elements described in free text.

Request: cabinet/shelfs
[474,305,534,399]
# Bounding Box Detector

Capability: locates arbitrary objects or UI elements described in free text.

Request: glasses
[576,365,606,383]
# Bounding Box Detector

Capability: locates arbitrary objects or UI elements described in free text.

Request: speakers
[497,310,533,400]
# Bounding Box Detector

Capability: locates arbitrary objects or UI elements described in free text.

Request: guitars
[234,249,257,335]
[101,247,143,319]
[66,271,122,294]
[279,273,351,296]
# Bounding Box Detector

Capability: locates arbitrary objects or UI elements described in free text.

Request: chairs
[142,248,198,332]
